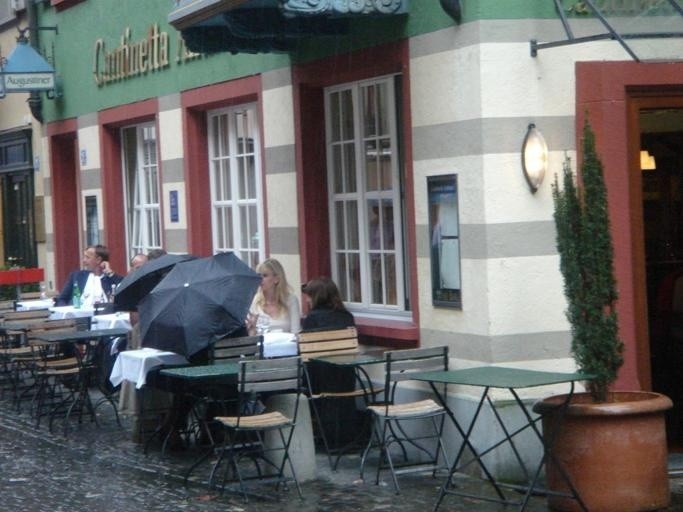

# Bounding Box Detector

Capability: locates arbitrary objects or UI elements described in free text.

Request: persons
[120,254,150,327]
[44,243,123,392]
[300,275,360,449]
[238,259,321,483]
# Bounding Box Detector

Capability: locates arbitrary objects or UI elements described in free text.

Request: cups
[39,281,47,300]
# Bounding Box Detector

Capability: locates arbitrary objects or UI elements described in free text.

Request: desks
[411,367,597,512]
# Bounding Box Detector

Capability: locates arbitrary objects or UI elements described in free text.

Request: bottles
[72,281,82,309]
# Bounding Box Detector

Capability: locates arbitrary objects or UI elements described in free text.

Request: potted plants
[531,107,673,512]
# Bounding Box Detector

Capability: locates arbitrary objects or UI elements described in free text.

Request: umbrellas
[109,251,193,313]
[133,247,262,361]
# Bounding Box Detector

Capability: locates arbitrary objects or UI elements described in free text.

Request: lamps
[521,122,549,194]
[0,25,59,100]
